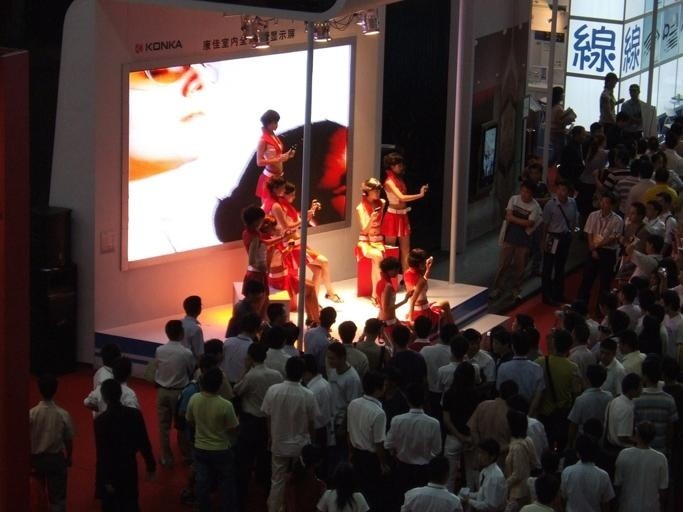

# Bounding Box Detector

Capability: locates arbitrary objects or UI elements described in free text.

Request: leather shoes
[543,295,564,306]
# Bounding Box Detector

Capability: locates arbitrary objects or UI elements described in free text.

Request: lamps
[314,21,332,43]
[244,17,270,49]
[357,9,380,36]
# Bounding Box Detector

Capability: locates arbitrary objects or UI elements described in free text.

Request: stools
[355,245,400,297]
[233,282,291,324]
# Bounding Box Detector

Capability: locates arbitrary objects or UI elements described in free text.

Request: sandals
[326,293,344,304]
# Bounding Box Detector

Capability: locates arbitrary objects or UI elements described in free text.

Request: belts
[383,319,396,326]
[359,236,383,242]
[283,239,301,247]
[247,265,265,273]
[269,269,288,279]
[263,169,284,179]
[413,304,428,311]
[387,207,412,215]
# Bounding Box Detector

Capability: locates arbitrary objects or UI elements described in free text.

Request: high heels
[306,319,332,333]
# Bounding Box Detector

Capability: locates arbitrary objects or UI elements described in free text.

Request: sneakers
[512,289,522,298]
[490,289,503,299]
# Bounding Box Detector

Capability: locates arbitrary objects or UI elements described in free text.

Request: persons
[84,277,683,510]
[241,176,343,331]
[27,373,75,512]
[373,249,454,332]
[256,110,296,204]
[357,152,428,301]
[494,73,683,322]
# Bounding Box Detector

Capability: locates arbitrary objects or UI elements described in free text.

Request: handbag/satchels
[574,235,591,264]
[551,408,571,443]
